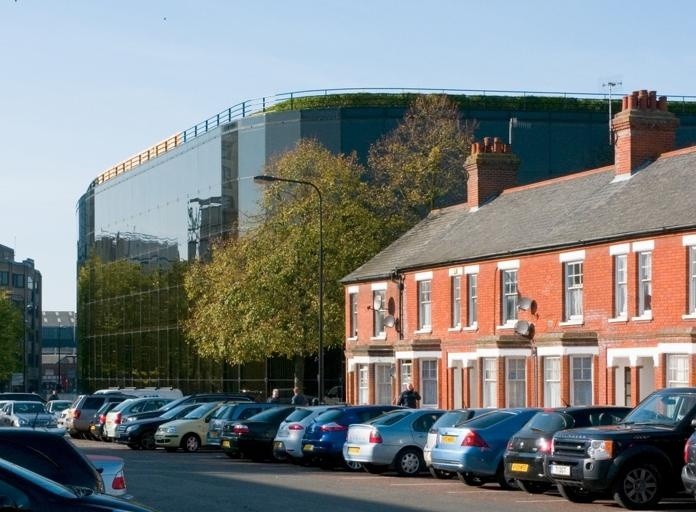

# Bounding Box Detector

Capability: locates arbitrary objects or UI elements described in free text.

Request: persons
[395,384,421,409]
[267,389,282,404]
[291,387,308,406]
[48,389,60,401]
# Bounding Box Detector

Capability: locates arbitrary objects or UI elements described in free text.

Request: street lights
[22,304,37,393]
[599,78,623,145]
[252,174,327,401]
[506,116,517,145]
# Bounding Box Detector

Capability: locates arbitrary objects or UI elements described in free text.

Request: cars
[680,430,696,495]
[85,454,127,496]
[272,405,356,468]
[502,396,679,504]
[430,406,552,488]
[0,457,156,512]
[0,392,83,439]
[301,405,404,473]
[114,404,203,451]
[154,400,257,454]
[344,407,449,476]
[420,407,505,478]
[219,405,294,463]
[205,404,277,447]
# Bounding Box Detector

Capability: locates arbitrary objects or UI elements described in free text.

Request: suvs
[66,392,138,441]
[88,402,120,443]
[103,396,176,442]
[541,387,696,510]
[120,392,256,423]
[0,425,105,494]
